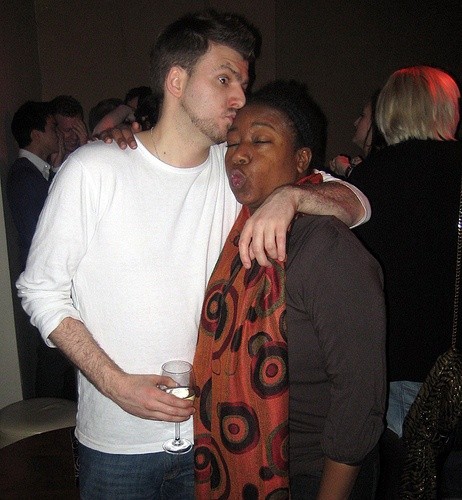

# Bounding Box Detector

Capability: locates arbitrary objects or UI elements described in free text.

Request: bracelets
[344,164,354,178]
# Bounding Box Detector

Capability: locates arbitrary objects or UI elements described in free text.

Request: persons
[16,83,378,409]
[95,79,387,500]
[344,68,461,498]
[14,5,372,498]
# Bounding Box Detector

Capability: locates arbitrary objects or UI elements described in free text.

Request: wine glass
[160,360,196,455]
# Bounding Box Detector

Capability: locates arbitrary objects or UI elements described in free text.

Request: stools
[0,396,77,447]
